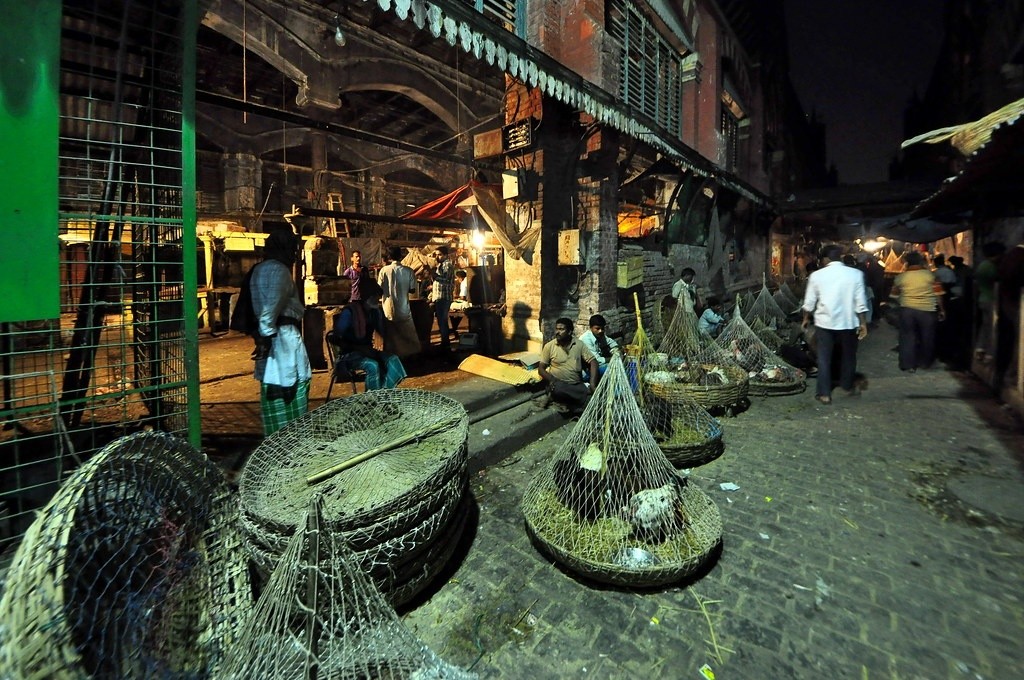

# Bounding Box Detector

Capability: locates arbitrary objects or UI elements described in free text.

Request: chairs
[325,331,366,402]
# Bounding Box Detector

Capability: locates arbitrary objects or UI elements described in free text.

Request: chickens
[554,338,803,539]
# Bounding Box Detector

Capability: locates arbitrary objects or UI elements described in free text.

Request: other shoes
[436,343,451,350]
[919,360,928,370]
[838,379,851,390]
[908,366,916,372]
[815,393,831,405]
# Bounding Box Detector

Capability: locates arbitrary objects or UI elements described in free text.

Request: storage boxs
[617,255,644,289]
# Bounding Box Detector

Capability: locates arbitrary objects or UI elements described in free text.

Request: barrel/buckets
[627,363,648,394]
[648,352,669,368]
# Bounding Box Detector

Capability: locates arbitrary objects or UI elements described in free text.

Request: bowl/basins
[609,548,663,568]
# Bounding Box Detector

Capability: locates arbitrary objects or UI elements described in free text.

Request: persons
[538,318,598,413]
[331,244,471,393]
[671,267,698,318]
[697,298,726,338]
[236,221,313,440]
[801,244,881,405]
[578,314,628,398]
[890,236,1024,399]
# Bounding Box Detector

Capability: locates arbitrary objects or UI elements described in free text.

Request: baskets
[644,363,751,408]
[1,429,251,679]
[599,418,725,467]
[748,361,805,396]
[233,386,468,620]
[521,460,723,588]
